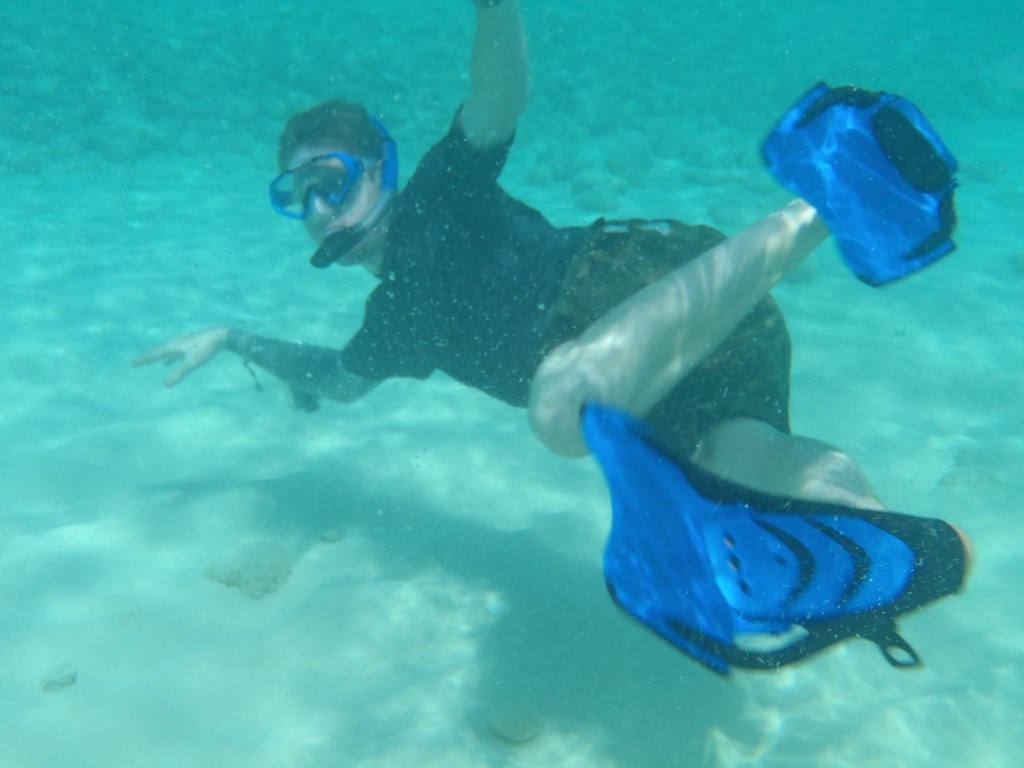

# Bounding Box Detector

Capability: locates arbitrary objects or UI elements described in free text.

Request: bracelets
[475,0,498,8]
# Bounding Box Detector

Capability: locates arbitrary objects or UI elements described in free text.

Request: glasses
[270,150,378,220]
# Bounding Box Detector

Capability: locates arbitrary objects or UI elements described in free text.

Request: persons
[130,0,970,678]
